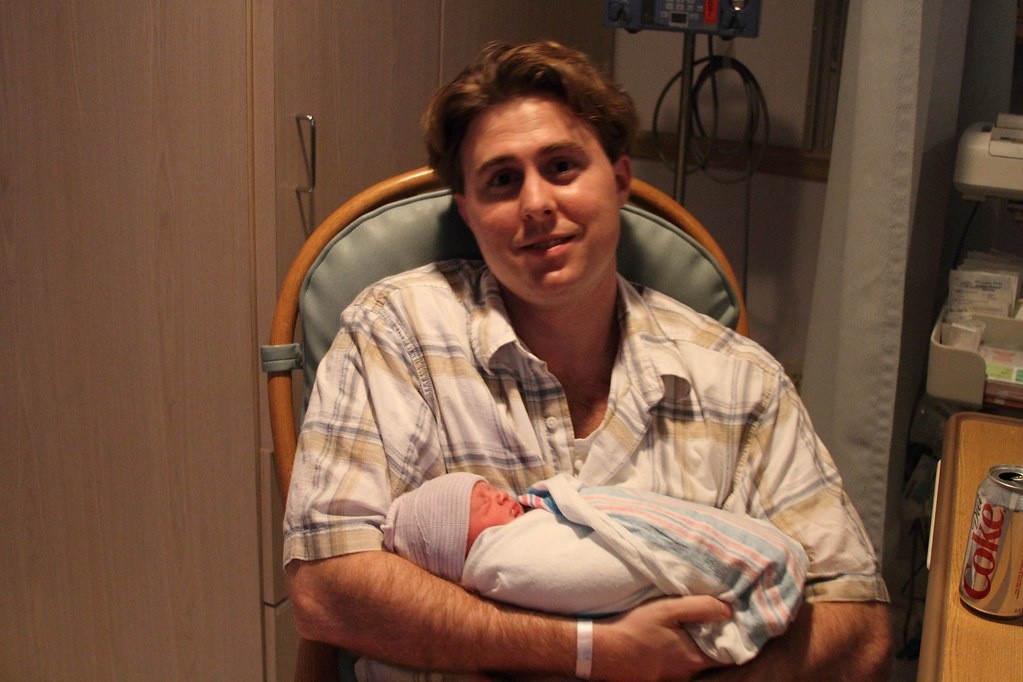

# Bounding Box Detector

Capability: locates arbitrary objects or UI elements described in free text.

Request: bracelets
[575,617,593,680]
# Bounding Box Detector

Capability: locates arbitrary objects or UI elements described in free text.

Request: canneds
[958,465,1023,620]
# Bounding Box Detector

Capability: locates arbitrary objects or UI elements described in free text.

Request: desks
[910,410,1023,682]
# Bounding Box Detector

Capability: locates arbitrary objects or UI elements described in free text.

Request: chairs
[258,162,749,681]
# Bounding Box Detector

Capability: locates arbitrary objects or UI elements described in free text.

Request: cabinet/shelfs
[875,0,1023,682]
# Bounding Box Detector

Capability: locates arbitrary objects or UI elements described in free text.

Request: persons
[282,41,892,682]
[380,472,809,666]
